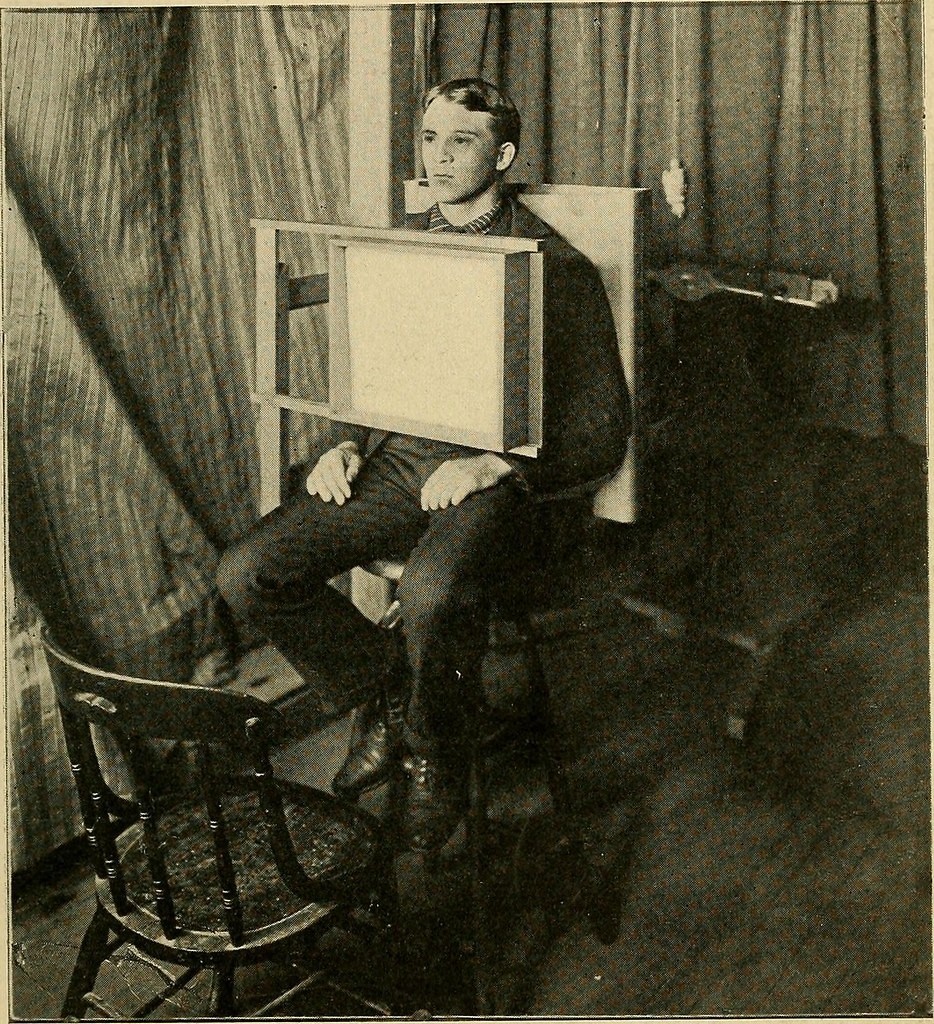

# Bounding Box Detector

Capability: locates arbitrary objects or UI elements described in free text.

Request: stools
[339,533,626,1016]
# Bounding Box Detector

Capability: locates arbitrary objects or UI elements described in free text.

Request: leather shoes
[333,666,414,796]
[396,701,488,855]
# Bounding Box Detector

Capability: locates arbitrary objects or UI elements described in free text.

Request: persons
[218,79,633,855]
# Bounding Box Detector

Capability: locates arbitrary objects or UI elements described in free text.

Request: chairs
[38,621,400,1018]
